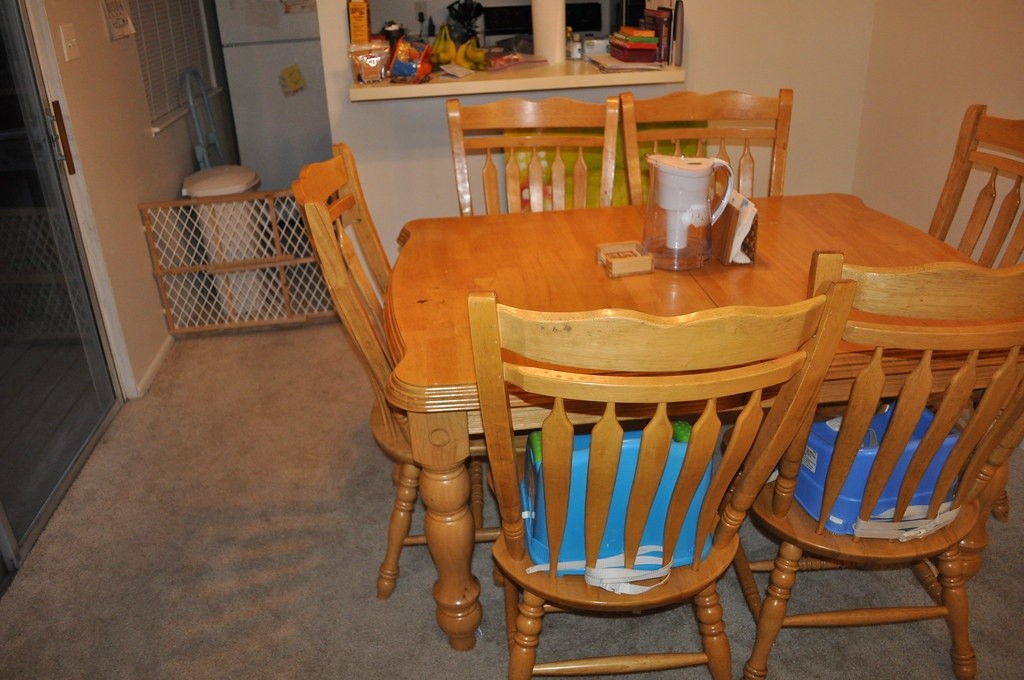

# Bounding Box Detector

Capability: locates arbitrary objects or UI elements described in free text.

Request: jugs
[642,152,735,272]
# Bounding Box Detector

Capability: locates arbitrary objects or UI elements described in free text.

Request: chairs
[718,251,1024,680]
[445,95,620,216]
[922,104,1023,522]
[292,143,504,600]
[620,88,794,204]
[467,280,858,680]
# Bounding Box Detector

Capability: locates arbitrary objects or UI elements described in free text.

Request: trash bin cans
[181,164,268,315]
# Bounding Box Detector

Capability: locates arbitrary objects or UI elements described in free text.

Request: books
[644,6,675,66]
[609,26,659,62]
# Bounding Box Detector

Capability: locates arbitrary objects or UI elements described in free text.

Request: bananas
[429,24,491,70]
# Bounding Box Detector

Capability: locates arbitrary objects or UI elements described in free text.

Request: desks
[378,193,1024,654]
